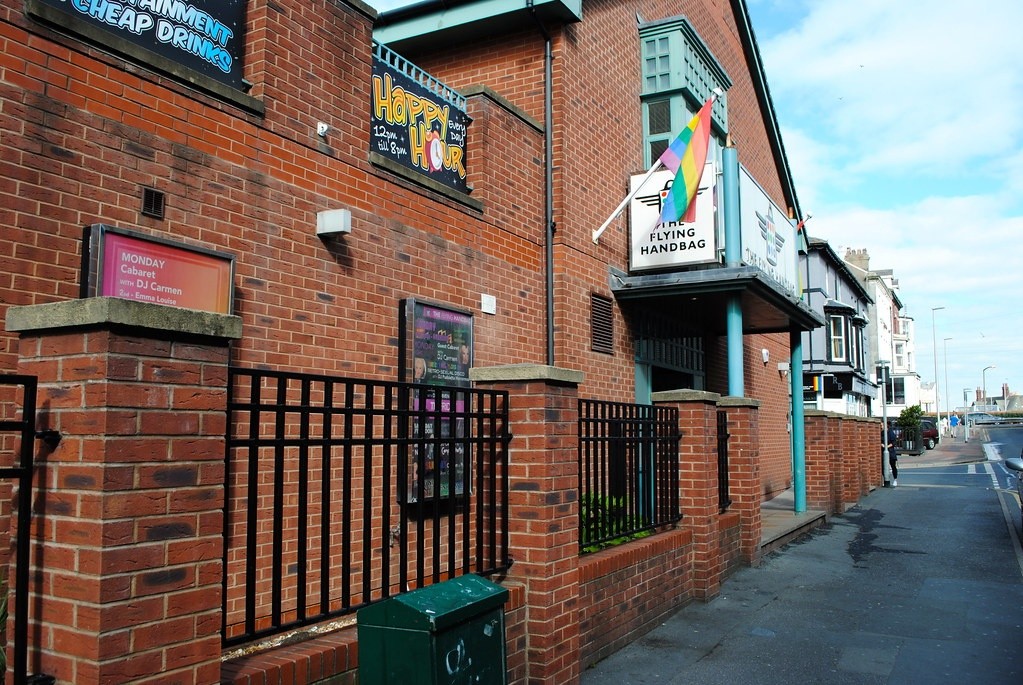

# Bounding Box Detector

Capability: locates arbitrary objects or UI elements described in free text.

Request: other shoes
[954,436,957,438]
[951,434,953,438]
[893,479,898,487]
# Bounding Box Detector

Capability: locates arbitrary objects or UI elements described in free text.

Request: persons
[880,421,898,487]
[950,411,961,438]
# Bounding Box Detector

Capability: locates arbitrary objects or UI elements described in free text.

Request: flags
[652,97,712,235]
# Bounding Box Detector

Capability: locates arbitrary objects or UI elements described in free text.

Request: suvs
[891,420,939,450]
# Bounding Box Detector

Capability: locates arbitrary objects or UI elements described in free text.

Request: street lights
[933,306,945,445]
[983,365,996,412]
[964,388,973,443]
[875,360,891,486]
[1004,377,1008,410]
[943,337,953,437]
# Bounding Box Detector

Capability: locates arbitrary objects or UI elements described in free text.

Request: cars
[1005,447,1023,514]
[961,412,1005,426]
